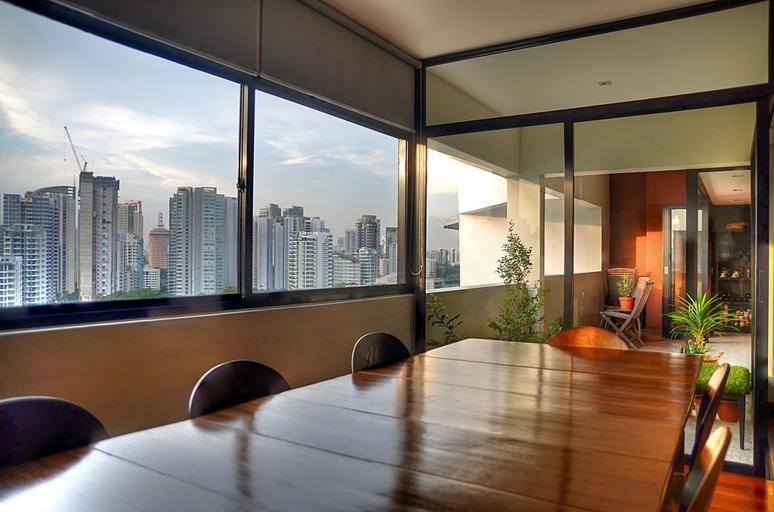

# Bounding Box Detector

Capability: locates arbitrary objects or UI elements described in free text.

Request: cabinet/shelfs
[715,228,755,333]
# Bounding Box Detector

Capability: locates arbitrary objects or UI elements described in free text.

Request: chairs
[678,359,734,467]
[663,424,732,511]
[1,393,114,468]
[348,329,416,374]
[599,266,656,351]
[186,357,293,419]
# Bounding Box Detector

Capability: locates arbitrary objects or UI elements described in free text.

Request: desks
[95,336,707,511]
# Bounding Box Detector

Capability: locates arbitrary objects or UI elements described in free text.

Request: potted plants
[654,286,751,423]
